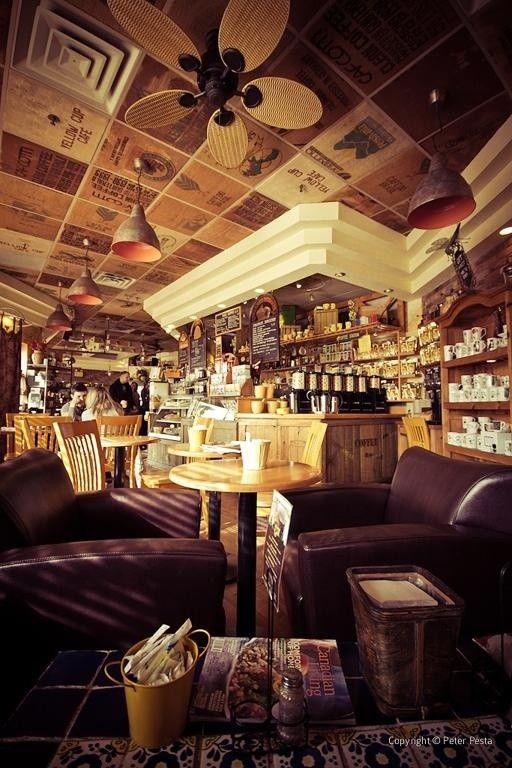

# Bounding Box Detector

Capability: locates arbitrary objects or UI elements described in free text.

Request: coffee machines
[288,367,388,413]
[423,366,443,425]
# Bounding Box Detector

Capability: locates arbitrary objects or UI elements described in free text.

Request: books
[189,635,358,727]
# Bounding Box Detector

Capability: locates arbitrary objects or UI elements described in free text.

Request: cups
[443,321,512,457]
[251,382,280,414]
[121,400,128,408]
[280,325,315,340]
[417,320,442,366]
[239,436,272,471]
[53,411,59,416]
[323,315,369,334]
[186,423,208,451]
[356,334,420,399]
[30,409,36,414]
[154,427,162,433]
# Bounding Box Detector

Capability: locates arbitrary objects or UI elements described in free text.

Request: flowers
[28,338,49,352]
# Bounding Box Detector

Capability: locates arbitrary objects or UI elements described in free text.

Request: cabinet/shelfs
[149,394,407,482]
[260,323,421,405]
[24,361,75,414]
[438,281,512,459]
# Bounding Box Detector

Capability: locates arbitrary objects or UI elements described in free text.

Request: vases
[30,350,43,364]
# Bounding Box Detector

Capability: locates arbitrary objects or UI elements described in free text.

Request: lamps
[111,158,164,271]
[61,237,103,310]
[403,89,478,235]
[40,296,76,332]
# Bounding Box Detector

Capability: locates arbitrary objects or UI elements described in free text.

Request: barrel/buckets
[187,425,207,449]
[104,627,211,747]
[239,438,271,470]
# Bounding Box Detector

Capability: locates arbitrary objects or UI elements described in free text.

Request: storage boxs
[359,304,388,325]
[280,325,300,337]
[311,308,338,334]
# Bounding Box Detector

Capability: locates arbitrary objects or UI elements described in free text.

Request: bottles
[276,664,308,747]
[176,365,209,395]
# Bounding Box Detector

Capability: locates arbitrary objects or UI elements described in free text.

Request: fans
[105,0,325,172]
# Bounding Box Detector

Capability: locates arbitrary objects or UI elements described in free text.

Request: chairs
[262,445,512,640]
[1,447,226,647]
[397,416,434,452]
[2,394,327,542]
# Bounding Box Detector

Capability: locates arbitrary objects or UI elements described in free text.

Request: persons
[109,371,133,415]
[81,386,125,435]
[59,383,87,422]
[130,381,140,415]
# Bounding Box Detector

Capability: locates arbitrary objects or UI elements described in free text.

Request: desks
[1,645,511,767]
